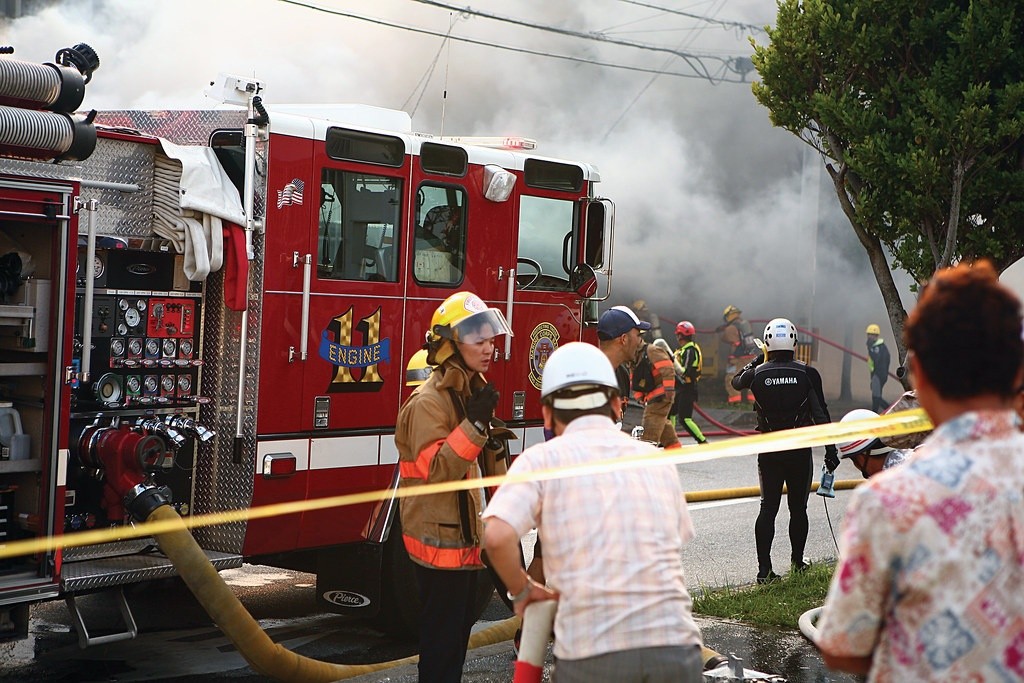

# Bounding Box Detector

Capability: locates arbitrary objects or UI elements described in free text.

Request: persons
[815,260,1024,683]
[483,342,704,683]
[633,301,661,345]
[654,321,707,445]
[732,319,841,586]
[865,323,890,413]
[630,329,676,448]
[513,305,651,657]
[394,289,520,683]
[716,306,757,407]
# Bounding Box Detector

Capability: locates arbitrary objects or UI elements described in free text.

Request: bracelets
[506,576,532,603]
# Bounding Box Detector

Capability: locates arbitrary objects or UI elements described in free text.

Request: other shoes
[791,561,809,573]
[756,571,782,584]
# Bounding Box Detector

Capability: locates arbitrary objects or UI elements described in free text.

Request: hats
[595,304,652,340]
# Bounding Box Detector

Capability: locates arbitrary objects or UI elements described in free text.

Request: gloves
[464,381,501,433]
[824,449,841,473]
[751,353,764,368]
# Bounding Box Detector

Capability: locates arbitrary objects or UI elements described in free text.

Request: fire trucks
[0,42,617,644]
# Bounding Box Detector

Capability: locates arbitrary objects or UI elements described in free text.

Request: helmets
[539,340,621,412]
[427,291,491,367]
[674,320,696,336]
[722,305,742,323]
[632,300,649,312]
[405,347,440,387]
[762,317,799,354]
[835,408,882,459]
[865,324,881,335]
